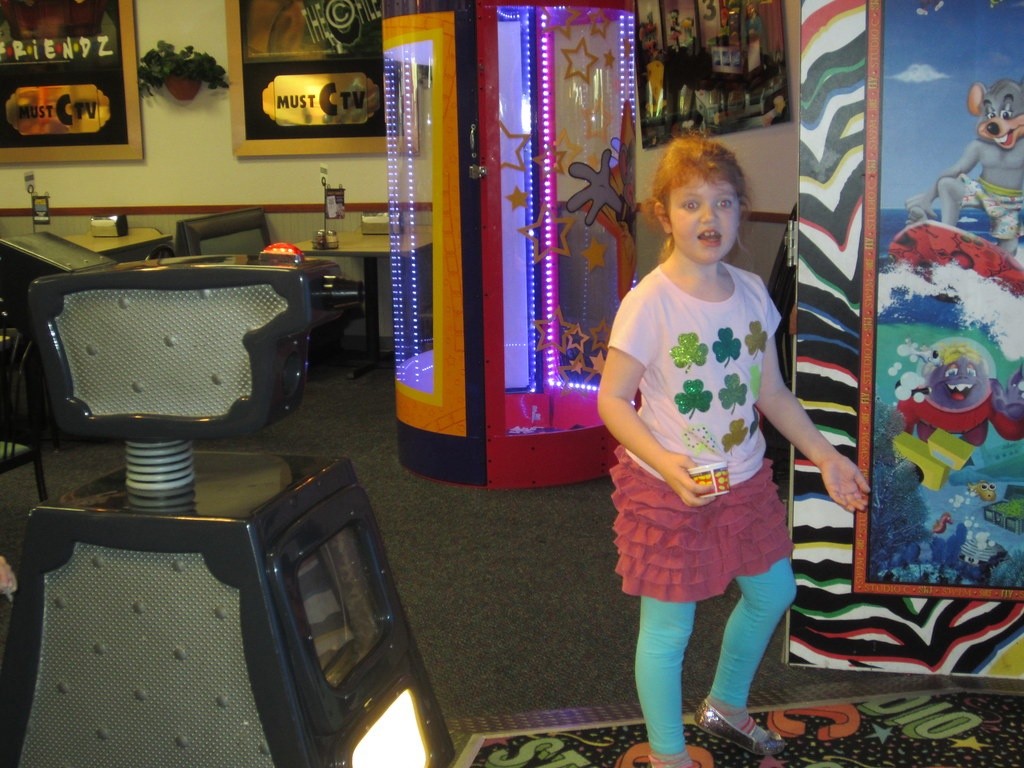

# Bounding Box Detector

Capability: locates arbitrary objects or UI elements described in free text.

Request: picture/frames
[0,0,143,164]
[224,0,420,157]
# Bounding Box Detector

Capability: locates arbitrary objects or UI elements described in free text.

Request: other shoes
[695,700,785,755]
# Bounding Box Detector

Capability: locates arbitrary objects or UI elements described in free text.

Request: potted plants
[138,39,232,101]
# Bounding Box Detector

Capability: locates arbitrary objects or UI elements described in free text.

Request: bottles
[313,229,339,249]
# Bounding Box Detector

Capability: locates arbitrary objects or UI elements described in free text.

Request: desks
[290,232,433,378]
[64,227,173,264]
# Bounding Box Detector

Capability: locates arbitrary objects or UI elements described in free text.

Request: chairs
[0,297,48,504]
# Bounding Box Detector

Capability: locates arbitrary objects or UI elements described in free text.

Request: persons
[597,136,871,768]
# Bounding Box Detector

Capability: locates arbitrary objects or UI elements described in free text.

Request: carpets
[442,688,1024,768]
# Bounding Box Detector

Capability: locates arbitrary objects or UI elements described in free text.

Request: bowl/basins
[687,461,730,499]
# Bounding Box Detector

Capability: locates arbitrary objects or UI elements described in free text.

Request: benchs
[175,207,260,256]
[184,209,365,383]
